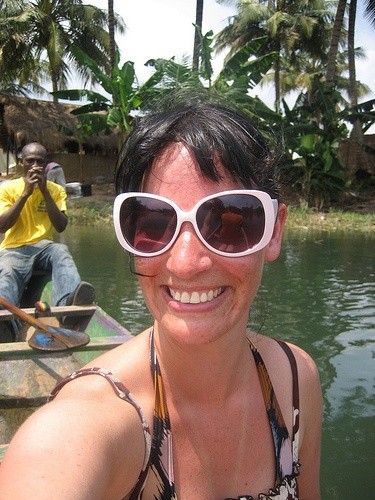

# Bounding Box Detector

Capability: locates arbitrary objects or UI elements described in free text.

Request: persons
[44,160,66,191]
[0,141,96,343]
[0,92,321,500]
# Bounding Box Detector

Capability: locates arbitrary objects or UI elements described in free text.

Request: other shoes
[0,307,16,343]
[61,282,96,332]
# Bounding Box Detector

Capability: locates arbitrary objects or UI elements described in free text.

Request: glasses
[112,190,279,258]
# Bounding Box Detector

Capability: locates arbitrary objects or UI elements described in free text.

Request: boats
[0,267,136,466]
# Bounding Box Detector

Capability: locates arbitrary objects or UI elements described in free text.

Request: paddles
[1,296,90,351]
